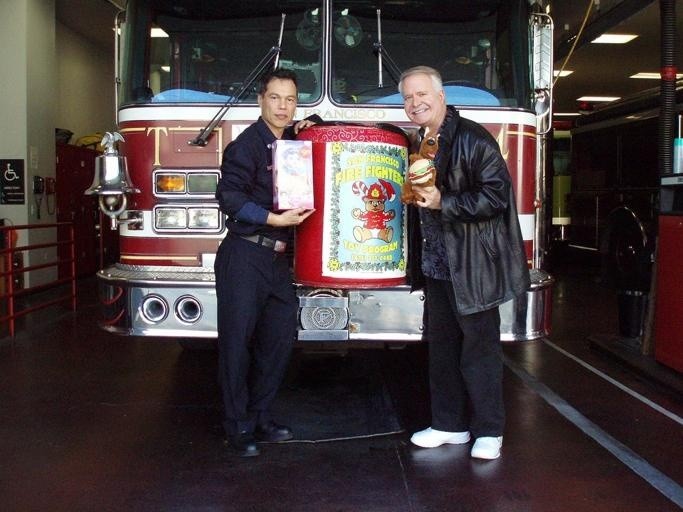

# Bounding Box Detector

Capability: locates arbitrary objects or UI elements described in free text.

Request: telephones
[46,176,55,195]
[34,176,44,195]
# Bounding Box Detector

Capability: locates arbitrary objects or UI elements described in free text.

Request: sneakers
[471,436,504,460]
[410,427,470,448]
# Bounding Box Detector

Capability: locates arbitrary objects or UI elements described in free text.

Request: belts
[240,232,287,253]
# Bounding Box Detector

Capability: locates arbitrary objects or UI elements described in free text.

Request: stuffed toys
[400,134,439,204]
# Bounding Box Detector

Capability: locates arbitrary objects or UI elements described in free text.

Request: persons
[212,68,333,462]
[397,66,530,461]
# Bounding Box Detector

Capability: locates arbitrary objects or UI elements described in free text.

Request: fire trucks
[86,0,556,346]
[562,79,683,331]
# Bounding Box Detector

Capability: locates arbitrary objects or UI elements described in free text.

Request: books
[270,137,316,211]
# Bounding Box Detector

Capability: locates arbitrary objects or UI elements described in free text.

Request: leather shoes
[225,433,260,458]
[255,420,294,443]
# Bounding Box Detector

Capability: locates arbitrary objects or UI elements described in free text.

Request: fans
[333,15,380,55]
[294,13,321,66]
